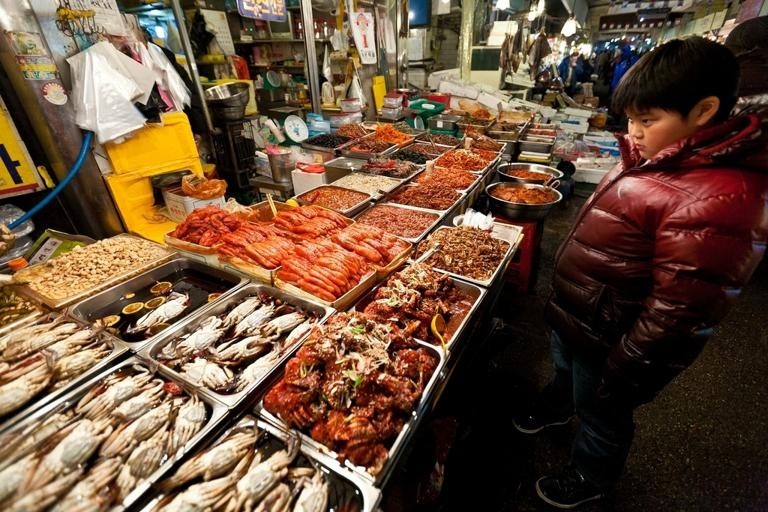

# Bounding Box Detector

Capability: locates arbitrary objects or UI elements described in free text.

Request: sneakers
[513,399,572,434]
[534,468,607,507]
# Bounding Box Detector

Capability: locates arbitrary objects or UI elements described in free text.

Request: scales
[269,106,309,147]
[255,63,281,90]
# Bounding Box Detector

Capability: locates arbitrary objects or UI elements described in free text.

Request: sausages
[217,205,408,301]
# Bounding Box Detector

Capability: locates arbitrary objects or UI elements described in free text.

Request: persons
[508,37,766,510]
[552,38,659,125]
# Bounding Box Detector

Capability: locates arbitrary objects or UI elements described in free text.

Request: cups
[266,150,295,184]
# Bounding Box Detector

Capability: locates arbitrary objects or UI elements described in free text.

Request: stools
[491,217,543,294]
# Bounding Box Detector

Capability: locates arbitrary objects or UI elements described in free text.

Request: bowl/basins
[203,82,250,119]
[496,162,564,182]
[486,181,563,218]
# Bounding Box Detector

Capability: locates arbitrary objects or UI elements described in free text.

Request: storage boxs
[161,187,227,225]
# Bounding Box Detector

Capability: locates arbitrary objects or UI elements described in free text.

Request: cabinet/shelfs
[225,1,359,120]
[218,116,267,206]
